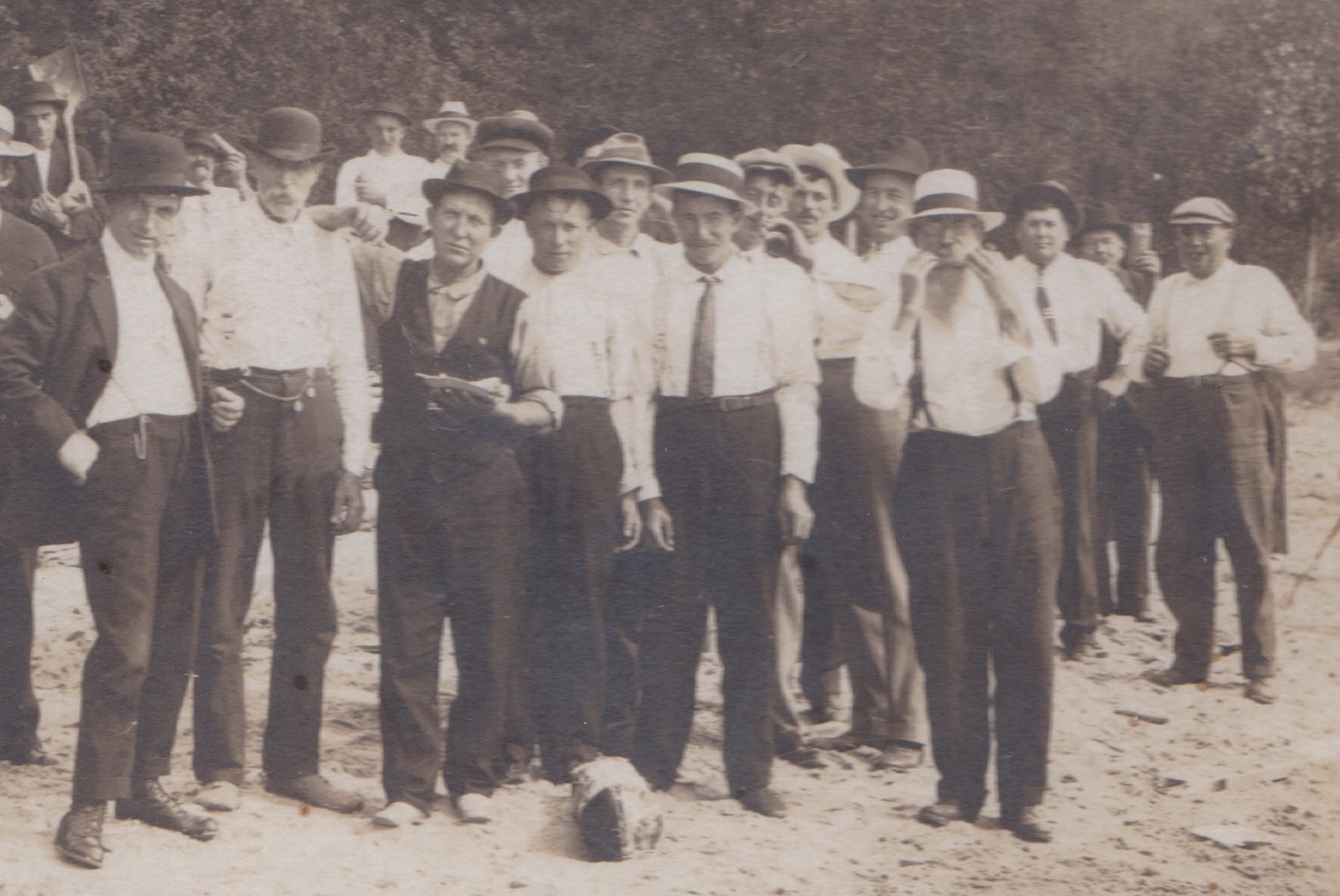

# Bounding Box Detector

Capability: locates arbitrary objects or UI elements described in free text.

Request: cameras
[764,228,792,257]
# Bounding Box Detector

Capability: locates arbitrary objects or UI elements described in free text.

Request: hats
[7,80,68,110]
[422,159,516,226]
[183,127,222,158]
[0,104,35,157]
[1073,202,1133,242]
[1022,179,1086,235]
[1166,196,1240,227]
[359,103,413,127]
[843,134,931,188]
[421,100,479,143]
[476,110,862,223]
[240,106,340,165]
[264,773,367,813]
[90,130,211,197]
[881,169,1006,236]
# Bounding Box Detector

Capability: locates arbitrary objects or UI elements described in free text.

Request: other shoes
[6,747,59,767]
[192,779,240,809]
[370,693,1059,845]
[1066,607,1278,704]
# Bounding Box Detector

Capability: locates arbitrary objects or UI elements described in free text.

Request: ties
[688,275,720,406]
[1036,263,1057,343]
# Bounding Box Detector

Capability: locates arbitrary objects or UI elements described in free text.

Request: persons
[155,123,255,276]
[504,165,646,787]
[0,83,106,260]
[850,169,1064,844]
[1005,179,1151,657]
[0,106,75,765]
[331,99,560,533]
[631,150,817,822]
[1133,196,1317,705]
[0,131,245,870]
[812,132,932,770]
[730,143,883,769]
[1074,199,1165,626]
[575,133,680,325]
[169,109,370,818]
[308,156,564,832]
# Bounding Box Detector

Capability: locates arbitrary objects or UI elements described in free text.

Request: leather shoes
[54,804,104,867]
[114,778,218,846]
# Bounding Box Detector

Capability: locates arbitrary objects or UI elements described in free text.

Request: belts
[663,392,777,412]
